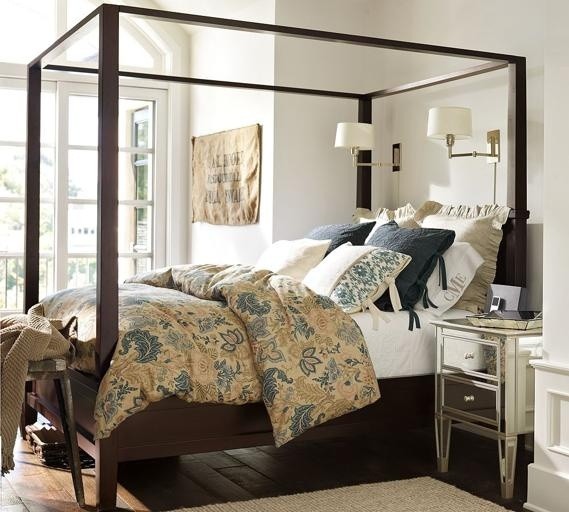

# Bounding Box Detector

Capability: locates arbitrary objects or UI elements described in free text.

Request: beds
[21,4,529,511]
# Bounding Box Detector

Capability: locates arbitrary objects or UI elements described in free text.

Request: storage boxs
[24,423,69,465]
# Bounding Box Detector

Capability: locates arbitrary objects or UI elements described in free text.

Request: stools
[26,358,90,508]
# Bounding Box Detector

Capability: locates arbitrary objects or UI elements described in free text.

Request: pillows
[360,220,456,332]
[350,203,420,246]
[303,221,377,261]
[412,200,512,314]
[363,207,486,317]
[301,241,413,315]
[255,240,331,281]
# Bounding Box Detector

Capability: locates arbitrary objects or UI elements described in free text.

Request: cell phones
[490,296,500,312]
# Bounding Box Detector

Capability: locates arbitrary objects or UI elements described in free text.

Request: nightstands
[431,316,545,500]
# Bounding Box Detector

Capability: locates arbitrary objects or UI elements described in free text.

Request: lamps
[425,106,501,165]
[334,122,401,172]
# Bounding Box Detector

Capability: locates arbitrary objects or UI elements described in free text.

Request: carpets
[171,474,515,511]
